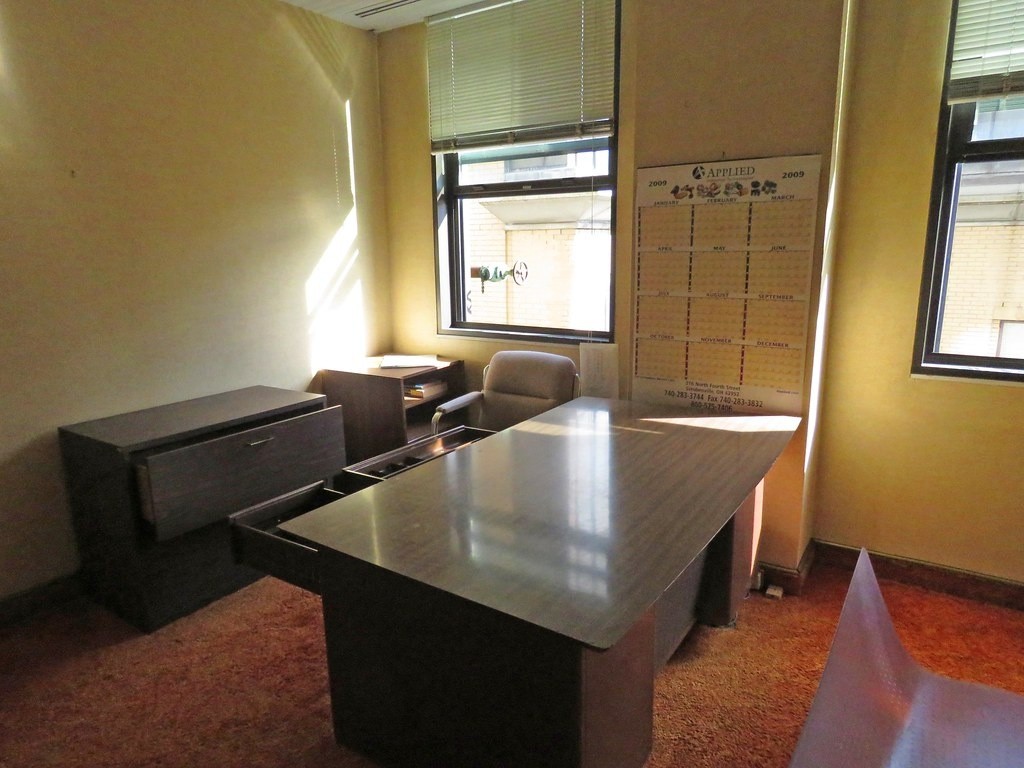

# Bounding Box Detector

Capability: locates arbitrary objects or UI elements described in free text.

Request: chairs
[431,350,581,437]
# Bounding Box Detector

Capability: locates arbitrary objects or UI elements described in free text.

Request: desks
[228,396,802,768]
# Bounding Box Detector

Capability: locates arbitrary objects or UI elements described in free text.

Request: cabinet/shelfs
[324,352,469,466]
[58,384,346,634]
